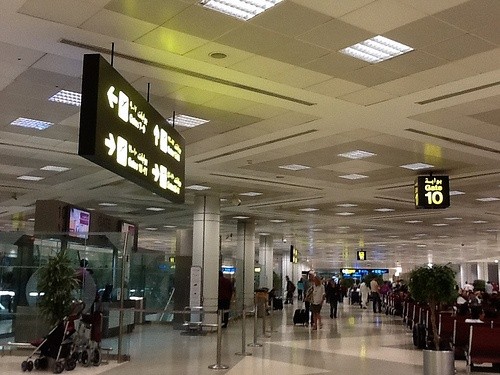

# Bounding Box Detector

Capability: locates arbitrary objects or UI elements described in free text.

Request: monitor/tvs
[66,206,92,239]
[117,219,138,246]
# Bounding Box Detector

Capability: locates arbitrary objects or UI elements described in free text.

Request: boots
[334,308,337,318]
[330,308,334,318]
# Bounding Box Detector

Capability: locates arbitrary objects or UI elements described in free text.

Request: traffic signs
[77,53,187,204]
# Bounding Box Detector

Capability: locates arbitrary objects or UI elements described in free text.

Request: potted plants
[403,260,460,375]
[12,238,82,343]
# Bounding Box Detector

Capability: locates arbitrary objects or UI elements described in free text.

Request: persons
[455,280,500,324]
[218,271,234,328]
[286,272,408,330]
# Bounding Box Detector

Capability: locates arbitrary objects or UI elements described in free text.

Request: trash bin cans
[254,289,265,317]
[129,296,146,324]
[422,349,455,375]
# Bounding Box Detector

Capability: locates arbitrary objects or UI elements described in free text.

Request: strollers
[20,299,102,374]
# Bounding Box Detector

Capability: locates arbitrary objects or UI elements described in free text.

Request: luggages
[413,308,428,348]
[293,301,307,325]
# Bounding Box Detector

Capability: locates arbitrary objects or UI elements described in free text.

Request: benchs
[382,290,500,375]
